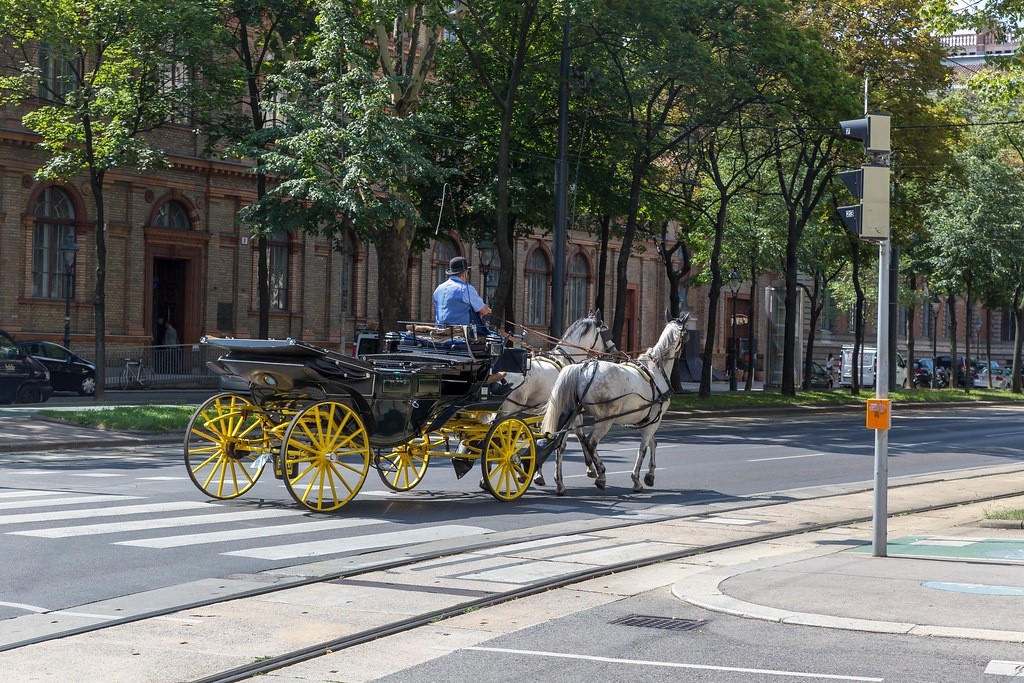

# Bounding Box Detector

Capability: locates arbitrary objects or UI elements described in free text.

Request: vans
[0,329,50,403]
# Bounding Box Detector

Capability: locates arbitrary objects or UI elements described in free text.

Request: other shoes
[485,370,508,384]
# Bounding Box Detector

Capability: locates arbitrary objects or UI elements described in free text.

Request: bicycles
[118,357,156,390]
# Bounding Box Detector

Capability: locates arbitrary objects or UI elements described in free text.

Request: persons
[825,353,835,379]
[158,321,181,374]
[433,257,514,400]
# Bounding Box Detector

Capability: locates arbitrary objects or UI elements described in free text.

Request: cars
[15,340,96,397]
[802,361,834,390]
[912,355,1013,389]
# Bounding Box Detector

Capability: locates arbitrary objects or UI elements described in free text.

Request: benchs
[400,323,491,356]
[363,352,473,373]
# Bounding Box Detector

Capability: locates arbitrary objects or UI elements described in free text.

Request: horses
[476,308,690,497]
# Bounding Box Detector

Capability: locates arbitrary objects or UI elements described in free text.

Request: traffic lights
[835,114,890,237]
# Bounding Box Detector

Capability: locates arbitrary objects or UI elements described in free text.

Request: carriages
[183,304,691,513]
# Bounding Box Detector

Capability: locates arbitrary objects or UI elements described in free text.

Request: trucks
[840,346,907,388]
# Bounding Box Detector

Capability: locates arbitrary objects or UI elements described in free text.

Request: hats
[445,256,471,275]
